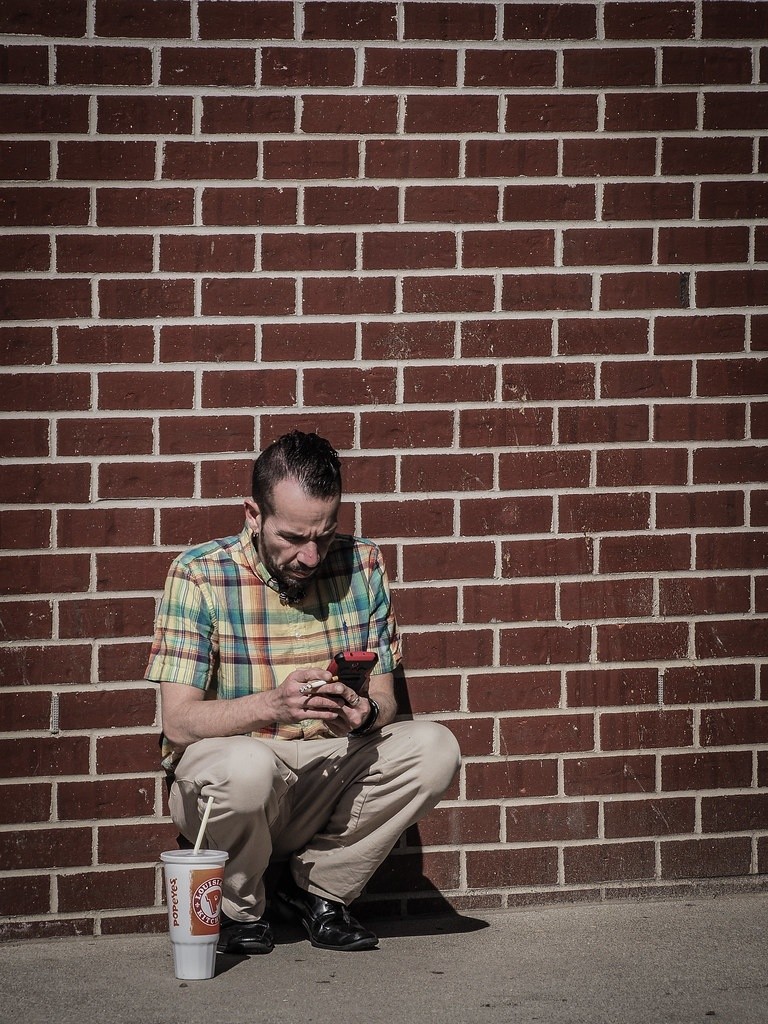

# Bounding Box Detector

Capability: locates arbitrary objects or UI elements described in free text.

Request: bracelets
[348,699,380,737]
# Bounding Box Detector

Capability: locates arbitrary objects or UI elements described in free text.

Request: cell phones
[327,652,379,696]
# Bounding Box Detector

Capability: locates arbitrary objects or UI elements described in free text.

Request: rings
[350,697,359,706]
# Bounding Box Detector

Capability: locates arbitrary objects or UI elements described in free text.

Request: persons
[143,432,463,954]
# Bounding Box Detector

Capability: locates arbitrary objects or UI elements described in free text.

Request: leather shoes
[216,910,274,954]
[270,880,378,951]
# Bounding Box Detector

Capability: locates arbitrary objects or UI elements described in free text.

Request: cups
[160,850,229,981]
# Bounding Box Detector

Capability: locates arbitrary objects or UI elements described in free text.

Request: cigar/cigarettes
[298,676,339,693]
